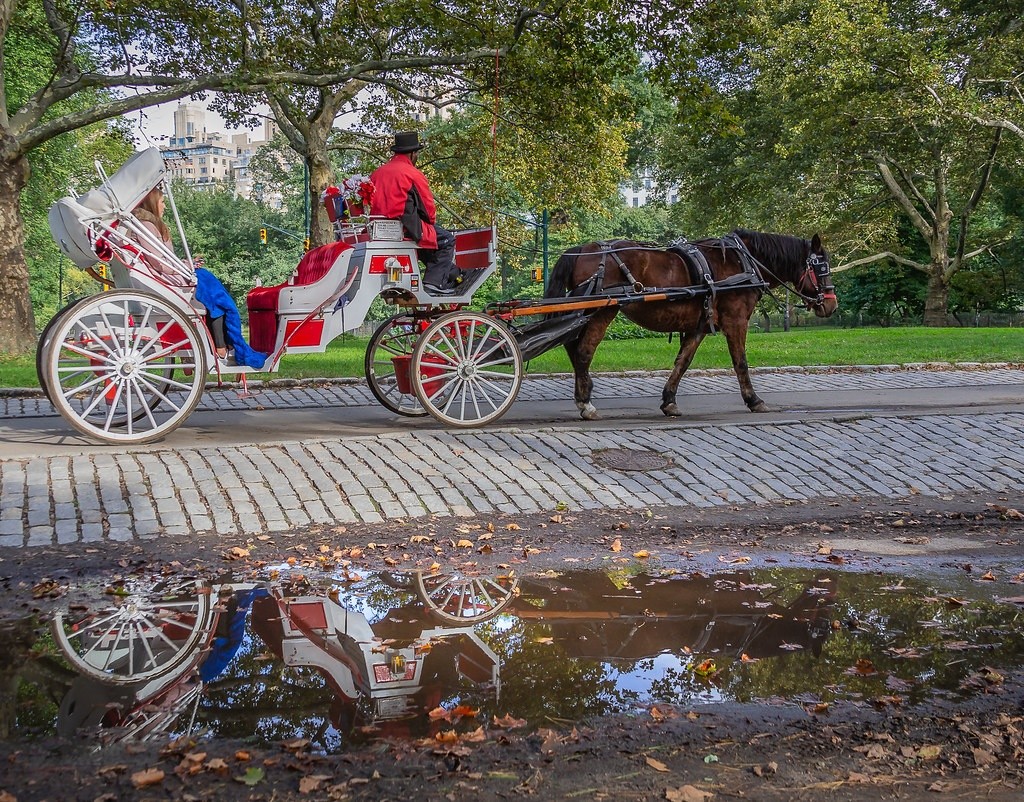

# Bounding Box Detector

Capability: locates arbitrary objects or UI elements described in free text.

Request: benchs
[343,232,430,251]
[109,247,207,358]
[251,595,364,705]
[246,242,356,354]
[57,613,207,744]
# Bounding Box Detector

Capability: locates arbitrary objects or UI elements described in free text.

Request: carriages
[63,571,841,739]
[34,147,841,447]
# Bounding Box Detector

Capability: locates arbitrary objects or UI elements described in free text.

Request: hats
[390,132,424,152]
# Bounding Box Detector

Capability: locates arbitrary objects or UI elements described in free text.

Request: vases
[345,197,365,217]
[323,197,339,222]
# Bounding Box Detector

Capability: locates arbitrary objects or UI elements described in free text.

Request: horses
[541,230,838,420]
[540,571,837,662]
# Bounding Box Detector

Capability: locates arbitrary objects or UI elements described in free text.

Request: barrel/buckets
[410,358,447,398]
[390,355,410,393]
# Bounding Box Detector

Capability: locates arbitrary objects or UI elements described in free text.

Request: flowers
[318,187,340,203]
[340,175,376,203]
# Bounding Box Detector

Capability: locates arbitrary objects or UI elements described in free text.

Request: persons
[362,130,464,296]
[328,675,442,750]
[133,187,239,365]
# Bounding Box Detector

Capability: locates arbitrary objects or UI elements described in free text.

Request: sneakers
[424,284,457,296]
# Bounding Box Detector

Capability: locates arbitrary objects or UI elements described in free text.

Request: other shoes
[216,349,237,365]
